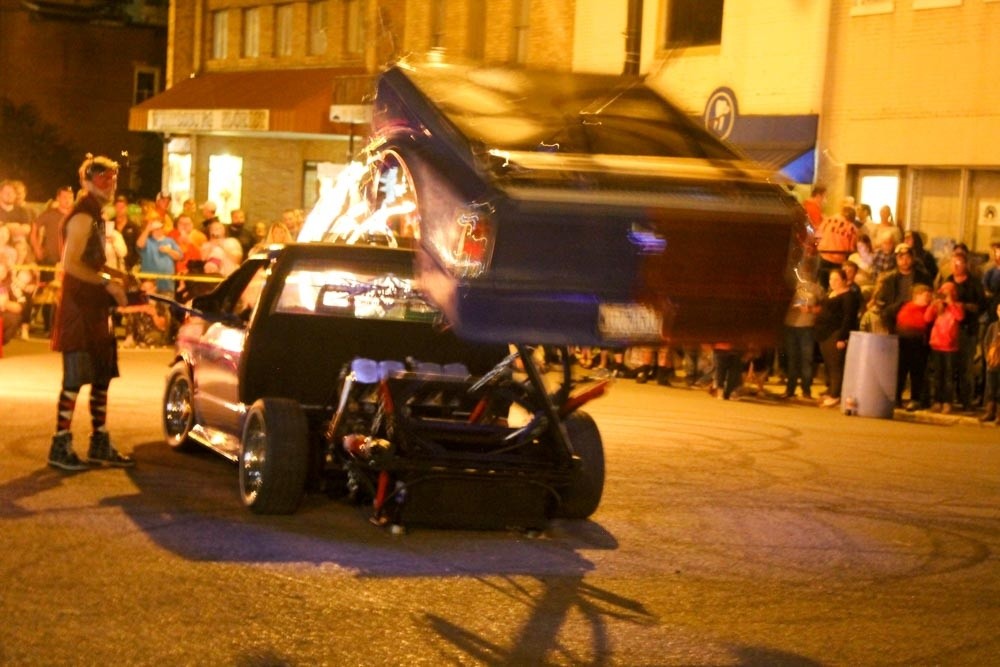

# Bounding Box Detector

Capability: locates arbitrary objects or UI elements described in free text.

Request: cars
[161,243,607,533]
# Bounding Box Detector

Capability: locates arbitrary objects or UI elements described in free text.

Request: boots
[48,432,87,470]
[88,434,134,466]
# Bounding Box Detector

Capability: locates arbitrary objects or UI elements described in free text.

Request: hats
[895,244,913,255]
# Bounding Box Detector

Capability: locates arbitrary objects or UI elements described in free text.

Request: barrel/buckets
[839,330,898,419]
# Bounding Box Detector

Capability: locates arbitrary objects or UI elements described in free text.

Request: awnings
[128,69,375,139]
[688,113,815,184]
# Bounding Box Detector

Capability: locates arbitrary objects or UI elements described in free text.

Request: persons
[507,186,999,426]
[0,178,305,349]
[48,155,138,472]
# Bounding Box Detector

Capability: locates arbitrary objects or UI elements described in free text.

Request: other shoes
[893,393,1000,427]
[591,364,839,411]
[17,316,176,349]
